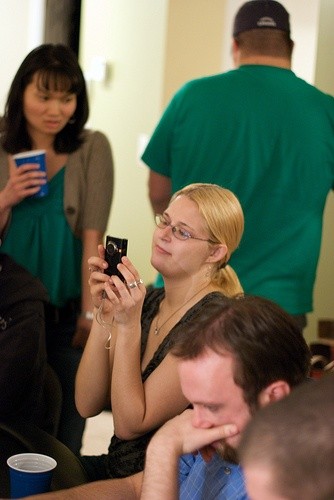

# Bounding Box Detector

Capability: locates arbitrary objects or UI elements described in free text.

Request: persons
[140,0,334,336]
[17,291,334,500]
[74,183,245,483]
[0,43,115,438]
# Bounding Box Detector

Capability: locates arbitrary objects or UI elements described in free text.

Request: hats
[232,0,290,35]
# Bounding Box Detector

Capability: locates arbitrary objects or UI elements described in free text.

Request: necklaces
[154,281,212,335]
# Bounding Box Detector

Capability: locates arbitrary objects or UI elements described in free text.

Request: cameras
[103,236,129,286]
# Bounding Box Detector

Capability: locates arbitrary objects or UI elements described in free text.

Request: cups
[14,148,49,200]
[7,452,58,499]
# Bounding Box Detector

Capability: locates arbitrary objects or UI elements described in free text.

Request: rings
[129,281,137,288]
[89,267,98,272]
[136,278,143,285]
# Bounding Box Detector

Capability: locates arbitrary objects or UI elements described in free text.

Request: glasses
[154,213,216,244]
